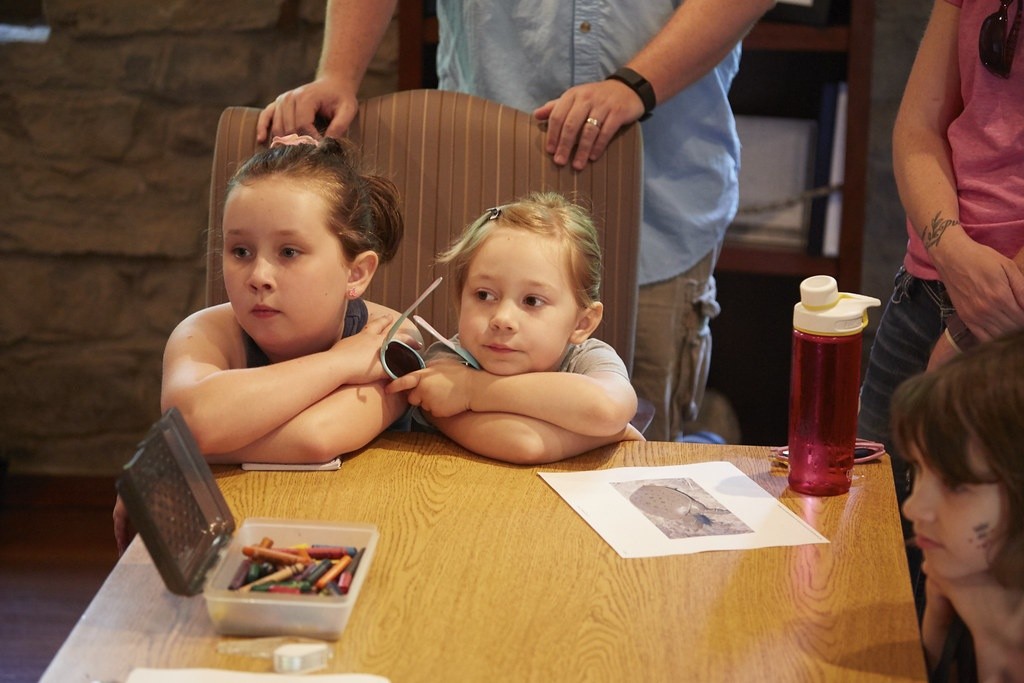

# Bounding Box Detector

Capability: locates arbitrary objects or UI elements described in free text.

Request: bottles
[786,273,882,497]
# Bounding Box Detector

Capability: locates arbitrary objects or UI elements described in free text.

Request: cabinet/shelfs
[710,0,872,445]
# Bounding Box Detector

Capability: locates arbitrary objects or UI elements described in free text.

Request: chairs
[209,88,643,380]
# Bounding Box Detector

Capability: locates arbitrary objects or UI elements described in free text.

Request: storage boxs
[199,517,380,641]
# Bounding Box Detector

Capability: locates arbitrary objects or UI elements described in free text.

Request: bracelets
[606,67,657,123]
[945,317,981,355]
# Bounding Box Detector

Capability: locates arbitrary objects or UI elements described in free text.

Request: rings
[585,118,601,128]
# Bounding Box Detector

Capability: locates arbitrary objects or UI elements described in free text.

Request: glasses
[978,0,1023,79]
[379,277,481,386]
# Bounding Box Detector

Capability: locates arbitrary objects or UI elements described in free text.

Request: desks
[42,432,928,683]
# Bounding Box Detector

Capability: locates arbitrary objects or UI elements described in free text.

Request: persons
[854,0,1024,495]
[158,132,429,467]
[257,0,813,442]
[891,325,1024,683]
[384,192,646,464]
